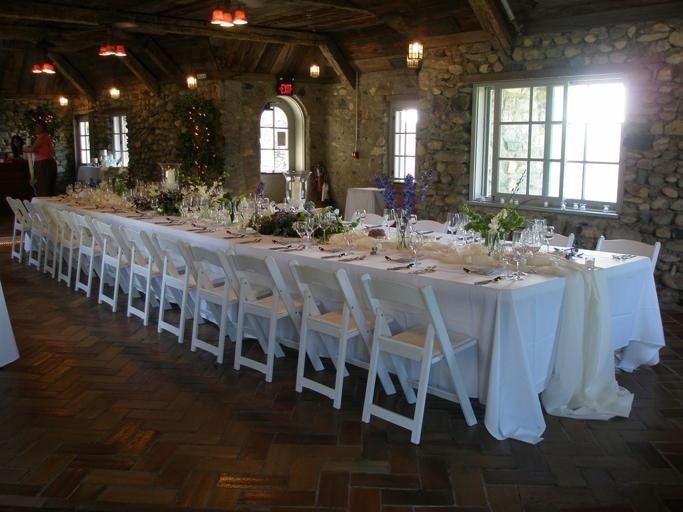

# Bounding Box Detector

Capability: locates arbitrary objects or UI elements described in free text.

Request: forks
[387,264,414,271]
[462,268,484,275]
[381,256,409,263]
[415,230,434,235]
[129,215,215,234]
[37,197,140,217]
[269,244,304,253]
[222,231,261,244]
[319,253,366,261]
[611,254,635,259]
[365,224,382,229]
[407,265,437,275]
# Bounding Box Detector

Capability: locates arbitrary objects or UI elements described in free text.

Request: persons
[21,119,57,196]
[10,133,29,161]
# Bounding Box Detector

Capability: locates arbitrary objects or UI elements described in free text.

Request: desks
[32,194,654,409]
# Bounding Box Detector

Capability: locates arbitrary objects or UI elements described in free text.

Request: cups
[281,170,312,208]
[157,161,181,190]
[585,257,595,270]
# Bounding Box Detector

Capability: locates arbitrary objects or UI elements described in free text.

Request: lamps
[307,27,321,80]
[207,0,248,31]
[405,19,425,71]
[29,22,56,78]
[184,38,199,91]
[96,8,126,59]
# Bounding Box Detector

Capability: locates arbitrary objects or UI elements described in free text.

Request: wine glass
[405,225,424,267]
[355,207,367,224]
[499,218,555,281]
[295,211,331,246]
[384,207,417,239]
[179,192,269,226]
[445,211,481,246]
[65,179,159,209]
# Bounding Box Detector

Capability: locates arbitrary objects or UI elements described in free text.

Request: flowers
[254,181,265,199]
[377,171,433,221]
[456,166,536,246]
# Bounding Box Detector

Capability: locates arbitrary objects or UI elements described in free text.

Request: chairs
[288,258,395,410]
[350,211,386,226]
[151,230,226,344]
[519,226,577,248]
[411,219,451,235]
[592,234,661,272]
[182,239,272,364]
[358,271,481,446]
[91,217,151,313]
[224,246,322,384]
[116,222,186,327]
[6,195,109,298]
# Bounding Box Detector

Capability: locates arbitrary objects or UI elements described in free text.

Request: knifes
[473,274,505,286]
